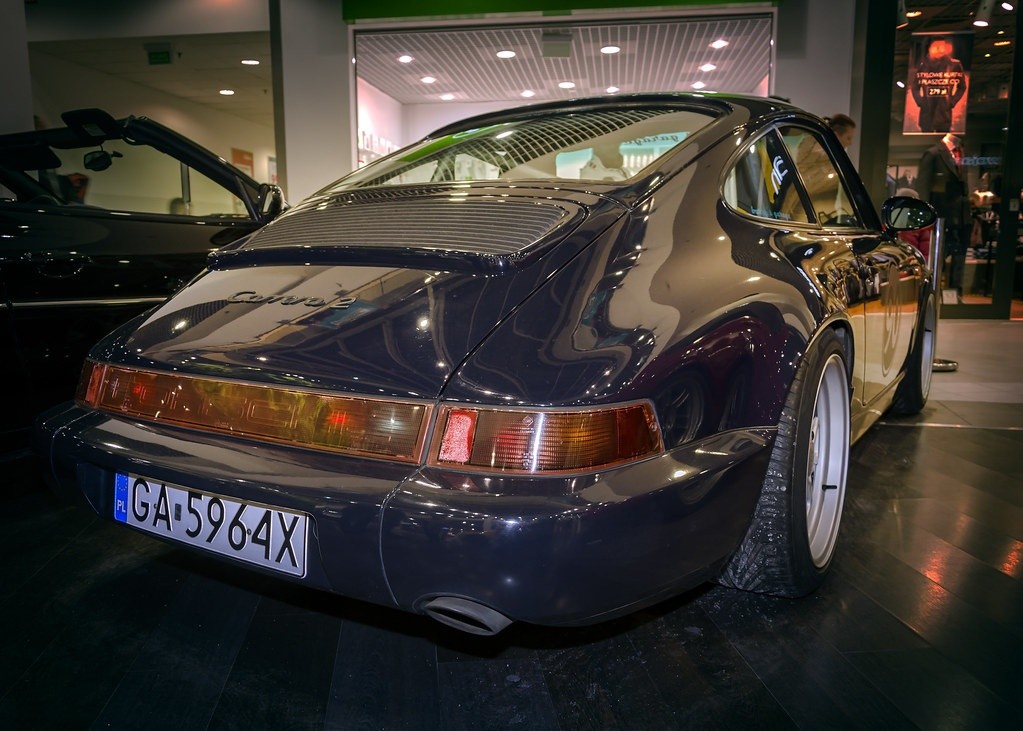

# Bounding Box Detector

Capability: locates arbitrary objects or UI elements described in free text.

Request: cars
[31,91,938,638]
[0,106,291,475]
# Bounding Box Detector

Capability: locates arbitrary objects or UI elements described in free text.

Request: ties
[954,146,962,170]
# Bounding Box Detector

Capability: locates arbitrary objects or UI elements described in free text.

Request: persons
[733,93,807,218]
[912,40,968,133]
[579,143,631,182]
[167,197,190,215]
[897,168,917,191]
[918,131,973,302]
[781,113,856,222]
